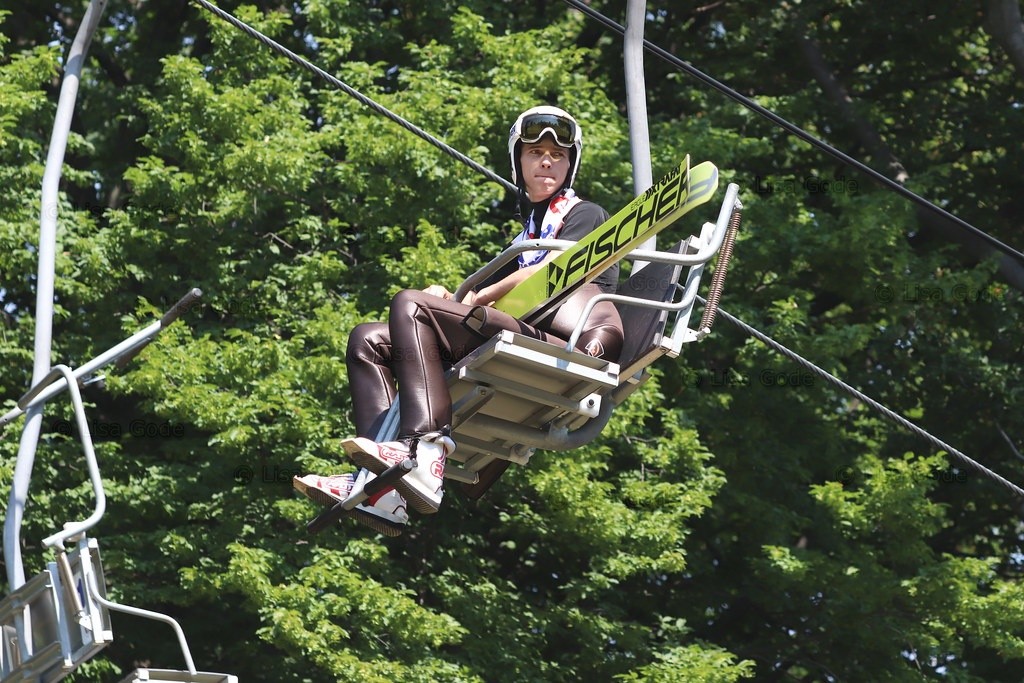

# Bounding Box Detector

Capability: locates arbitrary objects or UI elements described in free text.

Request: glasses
[520,113,576,144]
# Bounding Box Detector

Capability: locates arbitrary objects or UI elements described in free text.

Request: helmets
[508,105,582,189]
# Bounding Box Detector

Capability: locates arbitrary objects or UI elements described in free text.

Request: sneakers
[339,434,446,515]
[292,468,409,537]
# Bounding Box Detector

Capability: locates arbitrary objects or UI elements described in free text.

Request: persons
[294,105,619,537]
